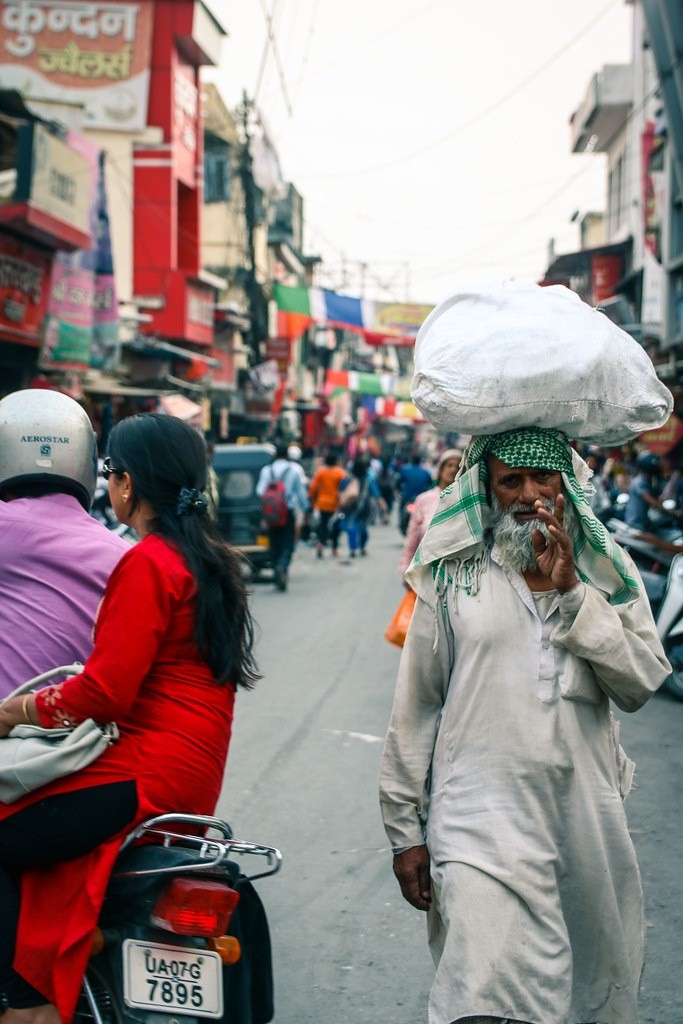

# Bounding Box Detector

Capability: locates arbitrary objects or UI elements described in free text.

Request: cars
[210,443,282,585]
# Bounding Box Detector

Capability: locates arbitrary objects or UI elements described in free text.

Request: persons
[304,442,445,558]
[255,442,312,592]
[379,427,673,1024]
[401,450,464,581]
[0,387,131,701]
[0,414,263,1024]
[573,436,683,574]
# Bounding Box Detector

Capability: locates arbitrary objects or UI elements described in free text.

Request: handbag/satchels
[1,664,118,806]
[384,589,417,648]
[335,476,360,508]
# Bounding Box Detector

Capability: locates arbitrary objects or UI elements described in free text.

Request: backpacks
[263,461,293,527]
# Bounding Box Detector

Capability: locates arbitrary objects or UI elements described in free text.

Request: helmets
[636,450,660,476]
[0,388,97,510]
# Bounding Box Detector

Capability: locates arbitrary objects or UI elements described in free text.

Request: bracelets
[23,694,34,724]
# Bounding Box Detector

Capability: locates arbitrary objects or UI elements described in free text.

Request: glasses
[101,456,125,481]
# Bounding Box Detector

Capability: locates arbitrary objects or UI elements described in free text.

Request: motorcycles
[593,493,630,534]
[399,494,417,536]
[606,499,683,701]
[0,813,281,1024]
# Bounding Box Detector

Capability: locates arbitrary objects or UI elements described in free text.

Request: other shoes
[381,516,389,524]
[274,565,287,590]
[361,548,366,555]
[332,550,339,556]
[350,548,355,558]
[317,543,323,557]
